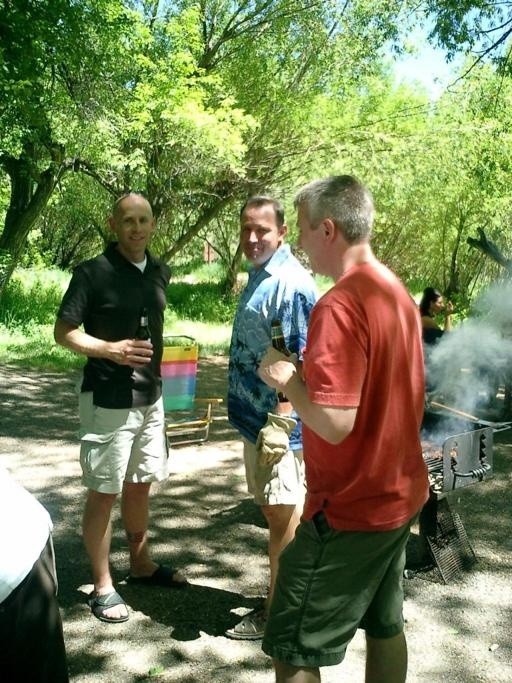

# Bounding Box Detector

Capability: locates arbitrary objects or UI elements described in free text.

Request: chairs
[161,334,223,446]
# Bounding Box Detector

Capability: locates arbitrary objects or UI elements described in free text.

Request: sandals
[223,609,265,641]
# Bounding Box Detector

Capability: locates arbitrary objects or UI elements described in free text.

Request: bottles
[131,306,152,356]
[269,319,295,403]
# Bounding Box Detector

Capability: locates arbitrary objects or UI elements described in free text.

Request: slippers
[87,588,130,625]
[130,562,191,587]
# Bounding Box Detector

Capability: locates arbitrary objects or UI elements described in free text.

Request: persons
[419,286,454,345]
[258,174,429,683]
[223,195,321,639]
[0,466,68,683]
[49,193,188,624]
